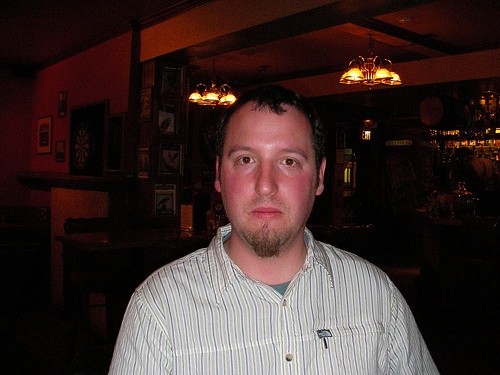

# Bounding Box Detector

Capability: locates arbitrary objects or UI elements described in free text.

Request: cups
[206,215,220,236]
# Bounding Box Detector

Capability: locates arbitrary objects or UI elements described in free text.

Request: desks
[55,226,217,365]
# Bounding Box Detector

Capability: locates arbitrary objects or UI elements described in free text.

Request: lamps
[188,55,238,108]
[339,35,402,86]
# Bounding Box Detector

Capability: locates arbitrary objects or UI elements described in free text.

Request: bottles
[429,127,500,164]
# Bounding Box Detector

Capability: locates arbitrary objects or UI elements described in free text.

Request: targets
[73,126,92,167]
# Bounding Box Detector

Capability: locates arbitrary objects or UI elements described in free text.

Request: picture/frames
[37,90,108,179]
[137,64,185,218]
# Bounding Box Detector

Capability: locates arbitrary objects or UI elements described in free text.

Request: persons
[108,85,439,375]
